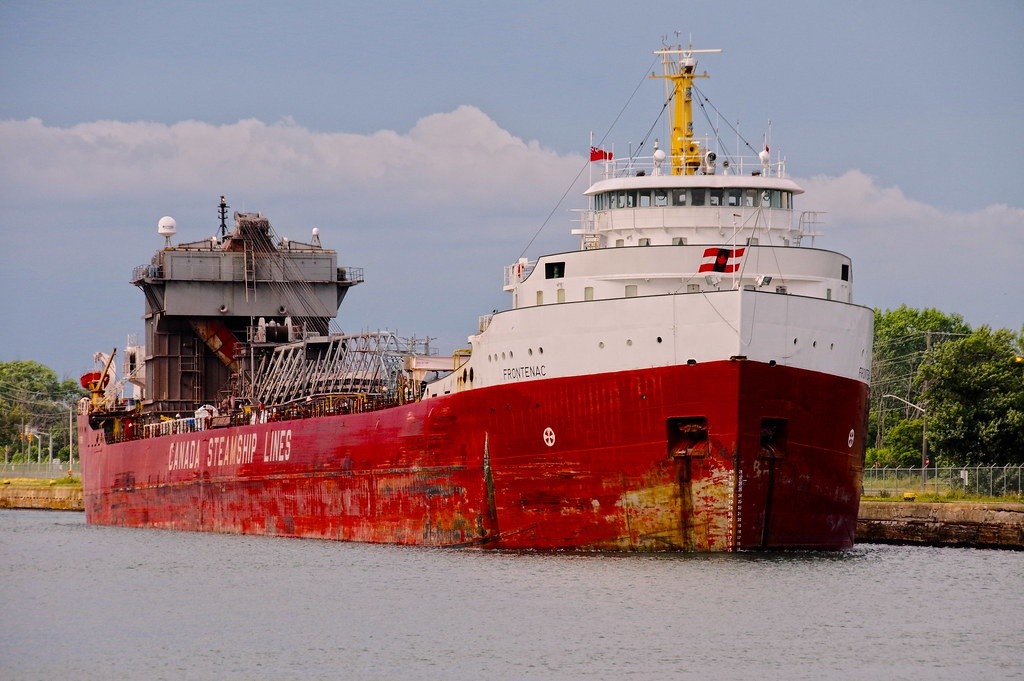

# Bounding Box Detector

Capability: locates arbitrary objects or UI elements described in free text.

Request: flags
[699,247,745,273]
[591,146,613,162]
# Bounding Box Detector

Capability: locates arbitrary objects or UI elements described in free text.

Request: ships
[73,28,883,557]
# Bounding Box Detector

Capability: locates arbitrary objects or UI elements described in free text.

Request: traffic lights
[19,432,24,443]
[28,433,33,442]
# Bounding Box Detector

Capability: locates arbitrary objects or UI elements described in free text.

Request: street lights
[883,394,927,495]
[55,401,74,478]
